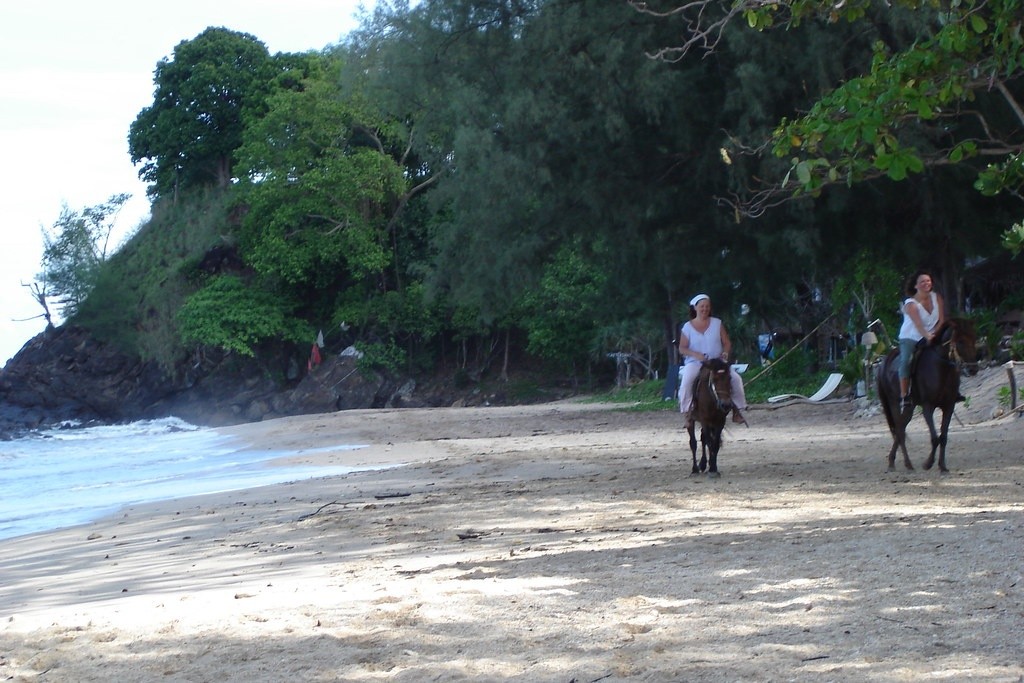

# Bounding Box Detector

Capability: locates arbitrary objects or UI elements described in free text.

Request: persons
[897,272,966,405]
[678,294,748,429]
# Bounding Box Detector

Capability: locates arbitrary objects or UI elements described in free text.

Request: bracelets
[722,352,729,360]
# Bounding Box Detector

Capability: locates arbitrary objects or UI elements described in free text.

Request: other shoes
[899,395,913,406]
[683,412,693,428]
[733,412,745,424]
[956,392,966,402]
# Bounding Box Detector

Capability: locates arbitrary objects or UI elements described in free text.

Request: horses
[686,358,735,478]
[875,316,980,476]
